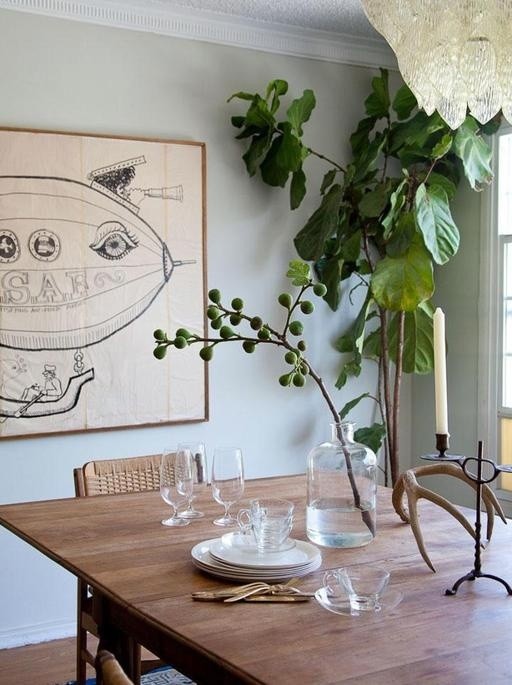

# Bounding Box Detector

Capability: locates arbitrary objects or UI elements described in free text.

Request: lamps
[360,0,512,130]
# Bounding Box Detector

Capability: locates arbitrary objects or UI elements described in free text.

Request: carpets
[57,664,197,685]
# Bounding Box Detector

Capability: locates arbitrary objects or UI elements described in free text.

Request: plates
[188,537,323,585]
[314,585,405,618]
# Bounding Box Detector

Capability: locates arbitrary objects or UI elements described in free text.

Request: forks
[190,581,318,603]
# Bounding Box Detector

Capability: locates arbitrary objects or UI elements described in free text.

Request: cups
[237,497,296,549]
[323,565,391,611]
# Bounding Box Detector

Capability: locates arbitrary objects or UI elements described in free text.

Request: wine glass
[159,449,191,527]
[211,446,245,527]
[174,441,206,519]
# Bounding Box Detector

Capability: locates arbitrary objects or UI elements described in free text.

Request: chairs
[73,452,204,685]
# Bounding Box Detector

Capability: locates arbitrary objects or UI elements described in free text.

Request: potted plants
[153,262,378,550]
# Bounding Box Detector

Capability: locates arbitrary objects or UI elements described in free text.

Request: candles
[433,305,449,439]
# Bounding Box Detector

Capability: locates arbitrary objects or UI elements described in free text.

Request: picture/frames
[0,127,209,439]
